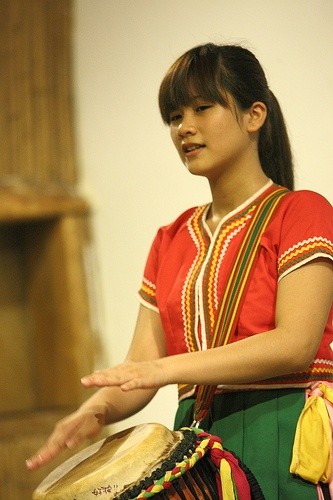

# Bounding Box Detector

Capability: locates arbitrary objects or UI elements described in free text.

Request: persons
[26,44,332,500]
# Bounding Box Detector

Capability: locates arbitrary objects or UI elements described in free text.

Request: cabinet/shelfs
[1,0,111,500]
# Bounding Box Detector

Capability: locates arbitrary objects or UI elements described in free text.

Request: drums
[31,422,216,500]
